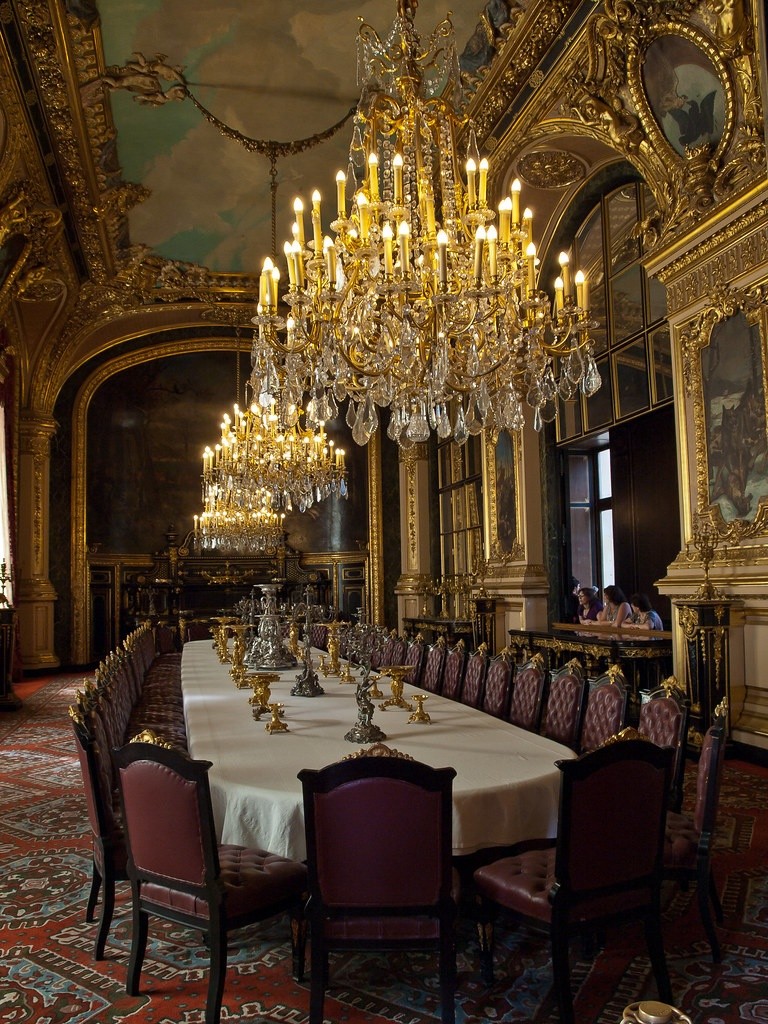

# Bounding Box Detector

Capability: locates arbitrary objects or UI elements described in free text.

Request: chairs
[67,618,729,976]
[110,728,296,1024]
[474,726,675,1024]
[296,738,485,1024]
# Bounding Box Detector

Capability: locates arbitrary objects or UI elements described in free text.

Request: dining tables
[180,636,579,858]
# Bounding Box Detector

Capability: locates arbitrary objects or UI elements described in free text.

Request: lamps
[193,329,349,554]
[247,0,602,450]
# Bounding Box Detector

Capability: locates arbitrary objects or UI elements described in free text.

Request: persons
[571,576,581,636]
[600,585,632,628]
[622,591,663,640]
[577,587,605,626]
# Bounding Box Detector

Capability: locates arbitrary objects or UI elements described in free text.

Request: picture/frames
[483,419,522,566]
[678,276,766,542]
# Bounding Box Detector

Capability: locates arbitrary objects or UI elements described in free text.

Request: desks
[508,622,673,722]
[402,617,476,651]
